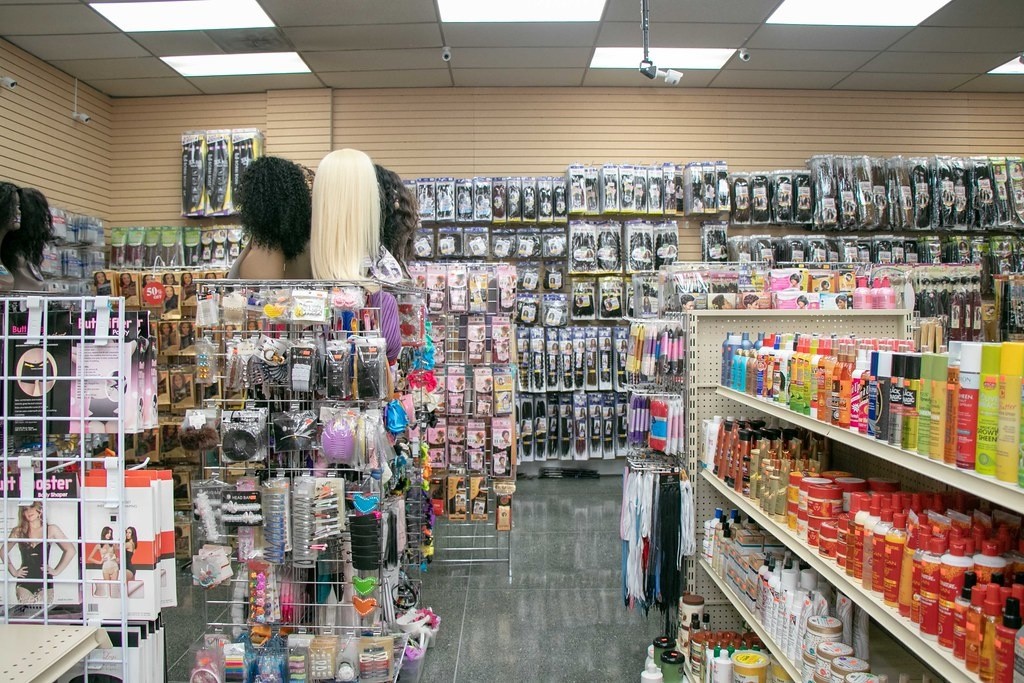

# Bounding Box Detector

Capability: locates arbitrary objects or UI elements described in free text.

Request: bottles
[688,613,768,683]
[714,329,1024,488]
[846,489,1024,683]
[708,507,757,579]
[756,551,820,663]
[641,664,663,683]
[645,645,654,668]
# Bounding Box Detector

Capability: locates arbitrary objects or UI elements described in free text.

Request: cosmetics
[640,329,1024,683]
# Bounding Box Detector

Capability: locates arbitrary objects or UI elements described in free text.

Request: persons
[523,277,531,286]
[643,297,651,313]
[88,526,137,581]
[203,231,250,258]
[94,272,258,552]
[549,277,556,288]
[21,349,55,396]
[0,502,77,603]
[412,274,516,514]
[681,270,854,309]
[229,148,417,282]
[576,298,583,307]
[606,302,612,311]
[547,313,554,325]
[523,338,628,349]
[523,404,627,434]
[418,244,676,259]
[0,181,55,291]
[523,310,528,320]
